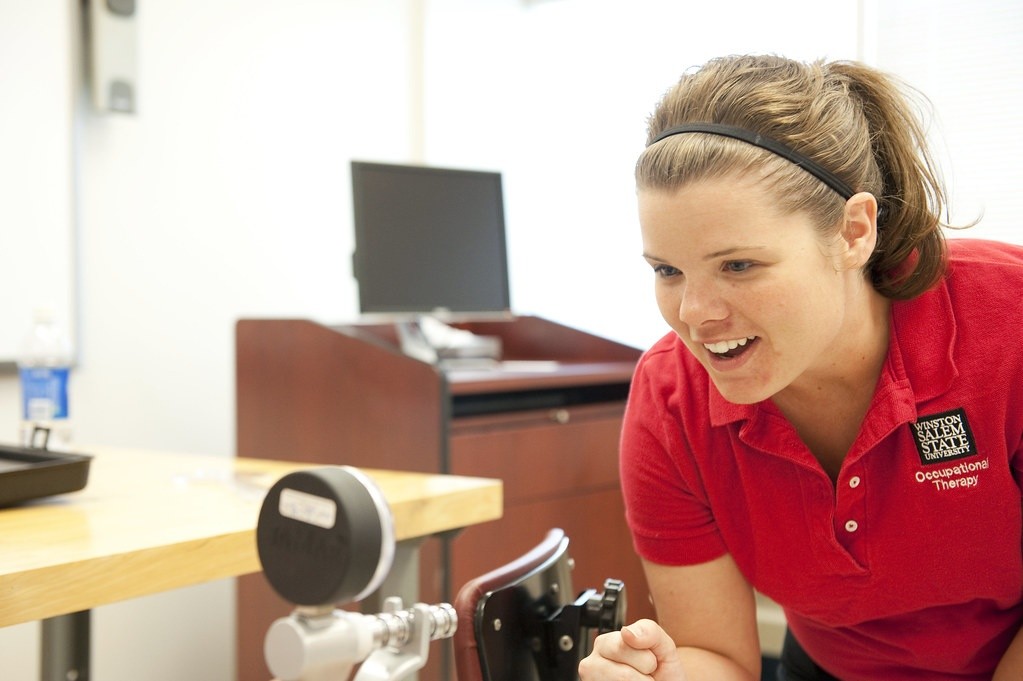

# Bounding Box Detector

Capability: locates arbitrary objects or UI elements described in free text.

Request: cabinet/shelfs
[236,316,660,680]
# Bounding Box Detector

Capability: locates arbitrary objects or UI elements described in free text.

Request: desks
[0,445,504,680]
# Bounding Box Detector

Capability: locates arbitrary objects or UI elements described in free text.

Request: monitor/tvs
[347,159,513,365]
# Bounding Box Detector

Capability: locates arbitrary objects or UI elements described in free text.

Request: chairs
[454,526,629,681]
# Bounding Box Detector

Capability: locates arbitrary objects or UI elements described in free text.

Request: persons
[578,56,1023,681]
